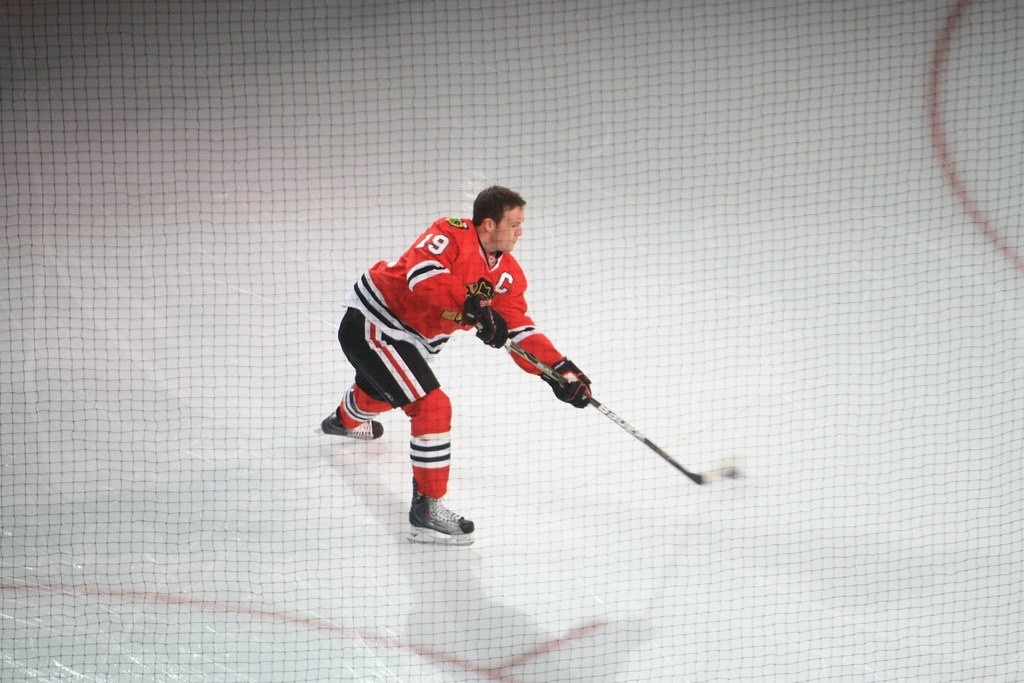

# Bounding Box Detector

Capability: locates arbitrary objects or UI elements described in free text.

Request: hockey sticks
[475,321,743,486]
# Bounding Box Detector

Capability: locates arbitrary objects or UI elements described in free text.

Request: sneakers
[321,406,384,440]
[407,476,474,545]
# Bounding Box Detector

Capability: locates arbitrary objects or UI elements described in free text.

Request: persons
[314,184,592,546]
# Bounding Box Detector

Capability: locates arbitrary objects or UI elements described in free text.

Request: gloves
[462,277,508,349]
[539,357,592,408]
